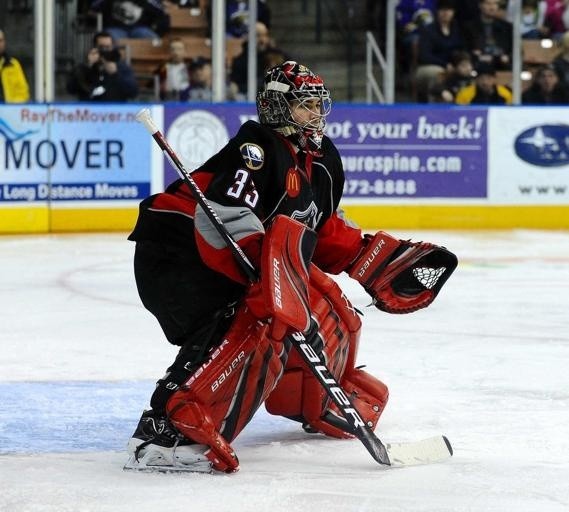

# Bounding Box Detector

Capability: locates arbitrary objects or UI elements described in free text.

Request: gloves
[244,273,290,341]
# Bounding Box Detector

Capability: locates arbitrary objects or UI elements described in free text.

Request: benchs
[493,38,562,97]
[102,0,244,102]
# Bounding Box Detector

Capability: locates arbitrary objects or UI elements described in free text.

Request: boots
[128,408,208,458]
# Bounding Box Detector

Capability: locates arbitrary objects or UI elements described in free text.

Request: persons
[126,61,458,473]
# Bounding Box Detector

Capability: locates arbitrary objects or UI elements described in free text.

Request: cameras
[99,47,121,62]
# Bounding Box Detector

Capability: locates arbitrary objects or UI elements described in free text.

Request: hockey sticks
[136,106,453,467]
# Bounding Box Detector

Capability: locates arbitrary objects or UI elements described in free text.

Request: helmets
[256,60,332,151]
